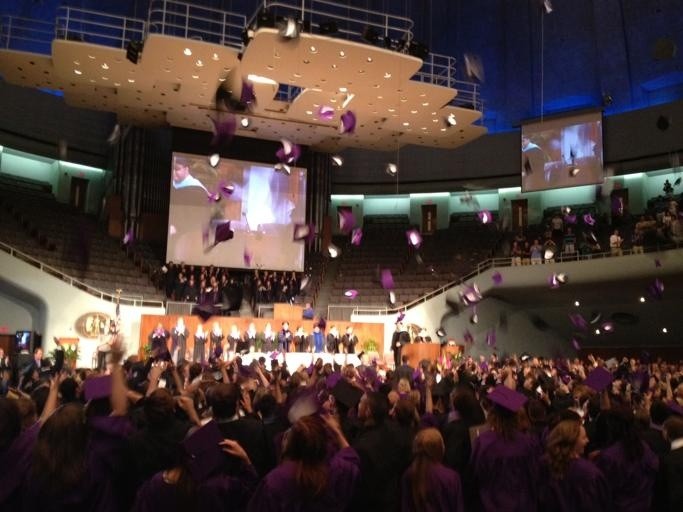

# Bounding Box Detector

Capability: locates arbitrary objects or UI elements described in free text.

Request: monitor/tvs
[15,330,42,353]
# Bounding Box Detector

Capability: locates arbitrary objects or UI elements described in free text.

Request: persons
[171,157,215,208]
[508,181,683,274]
[522,136,544,157]
[0,319,683,512]
[569,125,598,159]
[160,261,296,320]
